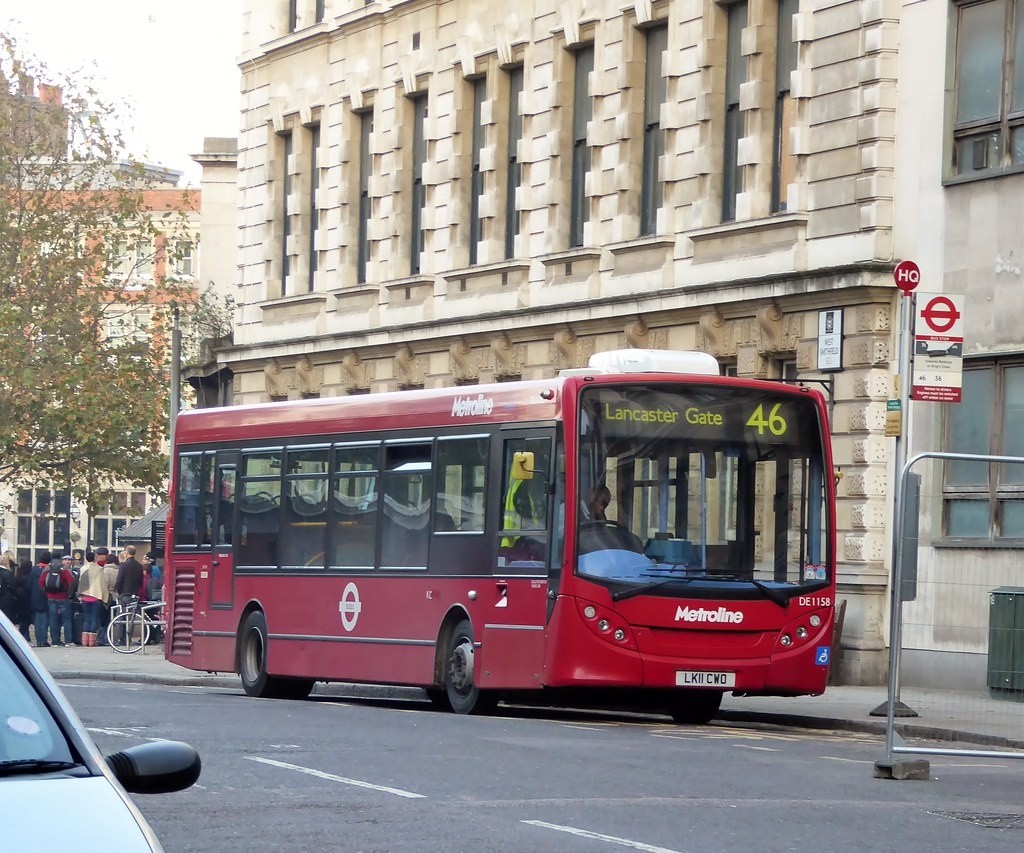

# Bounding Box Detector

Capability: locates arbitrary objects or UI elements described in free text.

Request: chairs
[173,491,540,568]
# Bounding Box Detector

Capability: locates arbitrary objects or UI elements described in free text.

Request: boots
[81,632,88,647]
[88,632,99,647]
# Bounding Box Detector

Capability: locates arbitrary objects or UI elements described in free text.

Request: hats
[144,552,156,563]
[62,555,73,560]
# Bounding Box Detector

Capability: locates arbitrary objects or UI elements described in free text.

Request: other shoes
[113,641,121,646]
[41,642,51,647]
[37,641,41,646]
[65,642,77,647]
[28,641,36,647]
[122,640,137,646]
[135,638,152,645]
[99,641,110,646]
[53,642,65,647]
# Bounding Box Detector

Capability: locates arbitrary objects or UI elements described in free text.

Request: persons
[0,545,161,648]
[557,485,611,548]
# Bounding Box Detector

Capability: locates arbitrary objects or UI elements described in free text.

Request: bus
[162,348,842,714]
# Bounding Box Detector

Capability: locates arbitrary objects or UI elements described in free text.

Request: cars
[0,610,201,853]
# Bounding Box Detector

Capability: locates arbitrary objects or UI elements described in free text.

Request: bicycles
[106,594,164,653]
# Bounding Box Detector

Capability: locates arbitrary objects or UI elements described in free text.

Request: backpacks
[44,563,68,593]
